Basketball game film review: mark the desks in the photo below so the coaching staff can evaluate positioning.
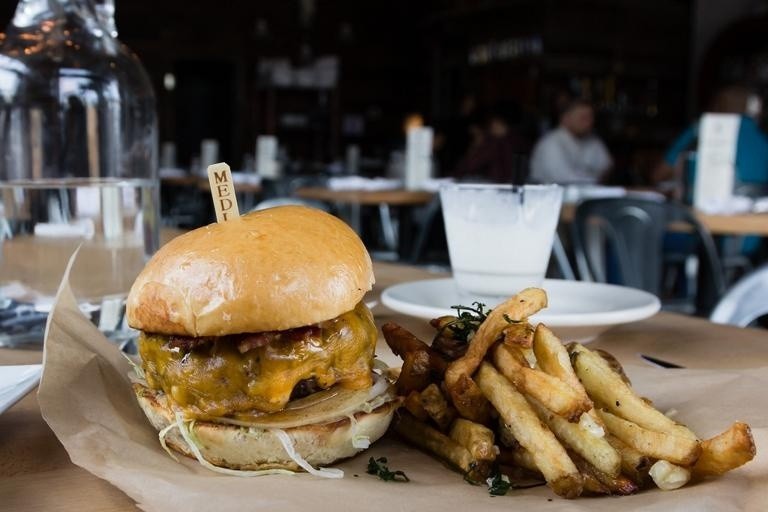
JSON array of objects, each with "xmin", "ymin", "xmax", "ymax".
[{"xmin": 0, "ymin": 172, "xmax": 768, "ymax": 512}]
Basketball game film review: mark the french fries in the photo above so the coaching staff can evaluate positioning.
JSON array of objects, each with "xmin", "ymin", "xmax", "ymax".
[{"xmin": 381, "ymin": 285, "xmax": 757, "ymax": 499}]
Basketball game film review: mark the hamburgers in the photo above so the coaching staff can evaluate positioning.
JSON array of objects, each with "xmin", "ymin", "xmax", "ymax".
[{"xmin": 126, "ymin": 161, "xmax": 394, "ymax": 471}]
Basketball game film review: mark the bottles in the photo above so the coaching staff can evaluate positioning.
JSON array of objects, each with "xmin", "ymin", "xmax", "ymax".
[{"xmin": 0, "ymin": 0, "xmax": 165, "ymax": 347}]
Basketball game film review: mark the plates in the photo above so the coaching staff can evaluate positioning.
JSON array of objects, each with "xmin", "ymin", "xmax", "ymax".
[{"xmin": 380, "ymin": 275, "xmax": 664, "ymax": 348}]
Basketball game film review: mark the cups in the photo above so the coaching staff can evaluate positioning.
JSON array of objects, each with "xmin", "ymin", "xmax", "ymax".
[{"xmin": 440, "ymin": 181, "xmax": 564, "ymax": 299}]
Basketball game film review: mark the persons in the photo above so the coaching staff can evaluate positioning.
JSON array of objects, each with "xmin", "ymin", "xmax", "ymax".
[
  {"xmin": 527, "ymin": 98, "xmax": 616, "ymax": 280},
  {"xmin": 654, "ymin": 87, "xmax": 768, "ymax": 320}
]
